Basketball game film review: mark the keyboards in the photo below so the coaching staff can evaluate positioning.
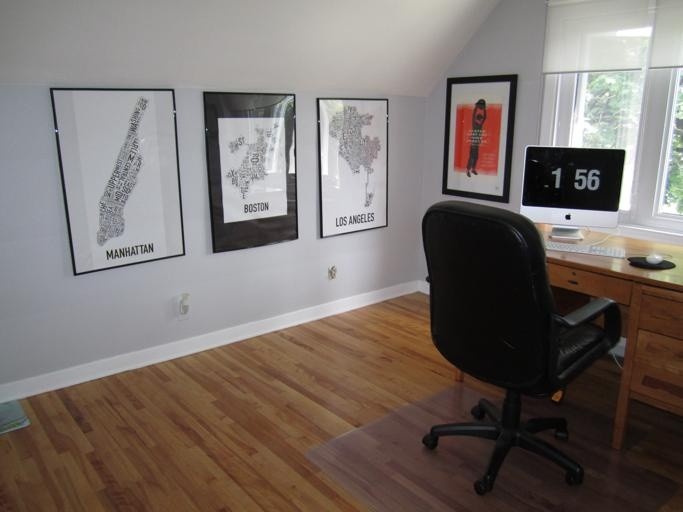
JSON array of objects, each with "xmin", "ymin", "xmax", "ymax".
[{"xmin": 542, "ymin": 240, "xmax": 626, "ymax": 260}]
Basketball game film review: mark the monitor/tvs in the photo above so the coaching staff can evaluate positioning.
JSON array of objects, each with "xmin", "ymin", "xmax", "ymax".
[{"xmin": 522, "ymin": 142, "xmax": 626, "ymax": 241}]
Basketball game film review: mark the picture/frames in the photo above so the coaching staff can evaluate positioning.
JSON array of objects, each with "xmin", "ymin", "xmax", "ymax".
[
  {"xmin": 317, "ymin": 98, "xmax": 389, "ymax": 239},
  {"xmin": 203, "ymin": 91, "xmax": 299, "ymax": 253},
  {"xmin": 49, "ymin": 87, "xmax": 186, "ymax": 275},
  {"xmin": 441, "ymin": 72, "xmax": 518, "ymax": 203}
]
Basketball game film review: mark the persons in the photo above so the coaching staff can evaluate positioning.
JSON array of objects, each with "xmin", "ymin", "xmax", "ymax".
[{"xmin": 466, "ymin": 99, "xmax": 487, "ymax": 177}]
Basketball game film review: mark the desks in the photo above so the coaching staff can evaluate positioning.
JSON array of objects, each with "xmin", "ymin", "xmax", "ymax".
[{"xmin": 530, "ymin": 221, "xmax": 683, "ymax": 452}]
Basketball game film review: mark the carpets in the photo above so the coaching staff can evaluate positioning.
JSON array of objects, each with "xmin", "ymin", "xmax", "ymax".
[{"xmin": 309, "ymin": 379, "xmax": 683, "ymax": 512}]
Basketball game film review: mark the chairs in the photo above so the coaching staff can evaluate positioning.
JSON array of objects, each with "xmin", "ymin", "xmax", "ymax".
[{"xmin": 423, "ymin": 200, "xmax": 622, "ymax": 496}]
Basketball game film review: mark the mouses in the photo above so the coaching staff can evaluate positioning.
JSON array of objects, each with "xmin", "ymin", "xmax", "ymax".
[{"xmin": 647, "ymin": 253, "xmax": 663, "ymax": 265}]
